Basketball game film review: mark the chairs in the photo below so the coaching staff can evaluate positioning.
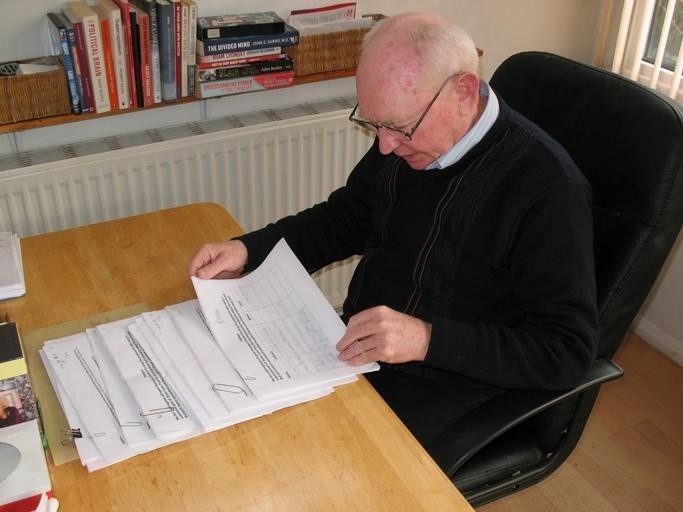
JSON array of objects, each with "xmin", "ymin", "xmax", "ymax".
[{"xmin": 308, "ymin": 52, "xmax": 683, "ymax": 508}]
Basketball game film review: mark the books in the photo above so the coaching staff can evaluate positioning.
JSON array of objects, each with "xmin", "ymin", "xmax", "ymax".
[
  {"xmin": 0, "ymin": 319, "xmax": 61, "ymax": 512},
  {"xmin": 46, "ymin": 2, "xmax": 301, "ymax": 117}
]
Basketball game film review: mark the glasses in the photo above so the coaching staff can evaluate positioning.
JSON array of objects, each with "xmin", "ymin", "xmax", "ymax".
[{"xmin": 348, "ymin": 70, "xmax": 464, "ymax": 146}]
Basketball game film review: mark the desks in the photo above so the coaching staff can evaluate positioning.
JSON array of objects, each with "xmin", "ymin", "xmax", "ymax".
[{"xmin": 0, "ymin": 204, "xmax": 477, "ymax": 512}]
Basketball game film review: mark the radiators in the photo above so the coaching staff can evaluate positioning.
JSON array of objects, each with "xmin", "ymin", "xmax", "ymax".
[{"xmin": 0, "ymin": 107, "xmax": 377, "ymax": 318}]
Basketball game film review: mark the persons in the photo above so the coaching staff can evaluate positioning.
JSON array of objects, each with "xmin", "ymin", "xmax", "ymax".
[{"xmin": 186, "ymin": 11, "xmax": 603, "ymax": 459}]
